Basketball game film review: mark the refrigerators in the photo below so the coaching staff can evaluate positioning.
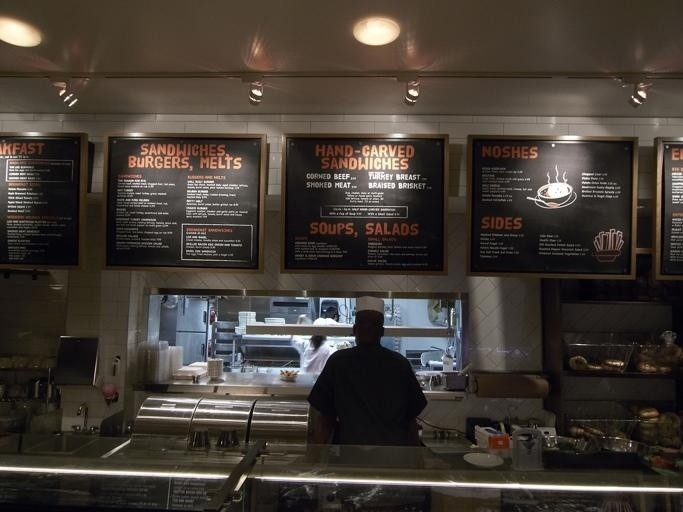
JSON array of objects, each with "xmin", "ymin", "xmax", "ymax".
[{"xmin": 174, "ymin": 299, "xmax": 212, "ymax": 365}]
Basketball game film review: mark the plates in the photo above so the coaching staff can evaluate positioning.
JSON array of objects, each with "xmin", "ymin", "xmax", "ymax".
[
  {"xmin": 208, "ymin": 359, "xmax": 224, "ymax": 380},
  {"xmin": 534, "ymin": 191, "xmax": 578, "ymax": 210},
  {"xmin": 463, "ymin": 452, "xmax": 504, "ymax": 469},
  {"xmin": 235, "ymin": 311, "xmax": 285, "ymax": 334}
]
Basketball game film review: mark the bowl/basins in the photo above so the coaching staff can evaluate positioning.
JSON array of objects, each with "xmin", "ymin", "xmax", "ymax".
[
  {"xmin": 537, "ymin": 183, "xmax": 573, "ymax": 205},
  {"xmin": 567, "ymin": 342, "xmax": 677, "ymax": 373},
  {"xmin": 280, "ymin": 372, "xmax": 297, "ymax": 381}
]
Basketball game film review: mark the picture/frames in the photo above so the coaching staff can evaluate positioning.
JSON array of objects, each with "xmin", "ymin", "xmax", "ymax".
[
  {"xmin": 466, "ymin": 133, "xmax": 639, "ymax": 278},
  {"xmin": 280, "ymin": 133, "xmax": 450, "ymax": 277},
  {"xmin": 650, "ymin": 137, "xmax": 683, "ymax": 281},
  {"xmin": 102, "ymin": 133, "xmax": 267, "ymax": 271},
  {"xmin": 0, "ymin": 131, "xmax": 91, "ymax": 270}
]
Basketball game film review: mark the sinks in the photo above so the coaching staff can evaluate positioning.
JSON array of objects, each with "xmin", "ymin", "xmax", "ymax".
[{"xmin": 22, "ymin": 433, "xmax": 97, "ymax": 457}]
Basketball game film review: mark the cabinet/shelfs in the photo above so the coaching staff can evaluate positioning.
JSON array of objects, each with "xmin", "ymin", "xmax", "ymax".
[
  {"xmin": 0, "ymin": 367, "xmax": 60, "ymax": 436},
  {"xmin": 539, "ymin": 277, "xmax": 683, "ymax": 434}
]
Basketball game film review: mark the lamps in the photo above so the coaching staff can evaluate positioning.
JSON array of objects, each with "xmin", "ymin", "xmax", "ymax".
[{"xmin": 0, "ymin": 69, "xmax": 683, "ymax": 108}]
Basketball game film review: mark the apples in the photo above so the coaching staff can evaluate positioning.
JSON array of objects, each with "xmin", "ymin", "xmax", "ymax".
[{"xmin": 101, "ymin": 383, "xmax": 115, "ymax": 397}]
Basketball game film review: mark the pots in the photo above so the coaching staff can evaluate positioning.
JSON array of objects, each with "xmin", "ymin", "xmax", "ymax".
[{"xmin": 541, "ymin": 426, "xmax": 647, "ymax": 463}]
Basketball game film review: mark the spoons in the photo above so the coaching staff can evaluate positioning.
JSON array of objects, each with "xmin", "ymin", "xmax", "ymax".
[{"xmin": 526, "ymin": 197, "xmax": 562, "ymax": 209}]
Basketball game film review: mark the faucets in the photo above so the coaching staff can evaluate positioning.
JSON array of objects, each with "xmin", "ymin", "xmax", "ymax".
[{"xmin": 77, "ymin": 403, "xmax": 89, "ymax": 433}]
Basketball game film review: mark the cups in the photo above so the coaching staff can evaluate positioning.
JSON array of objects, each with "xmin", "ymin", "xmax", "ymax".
[
  {"xmin": 216, "ymin": 426, "xmax": 240, "ymax": 449},
  {"xmin": 447, "ymin": 307, "xmax": 456, "ymax": 328},
  {"xmin": 188, "ymin": 426, "xmax": 211, "ymax": 450}
]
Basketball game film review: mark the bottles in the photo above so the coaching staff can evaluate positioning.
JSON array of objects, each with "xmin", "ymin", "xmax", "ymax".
[{"xmin": 442, "ymin": 342, "xmax": 456, "ymax": 372}]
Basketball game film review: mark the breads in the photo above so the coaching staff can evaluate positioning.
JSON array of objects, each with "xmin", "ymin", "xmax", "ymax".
[
  {"xmin": 569, "ymin": 344, "xmax": 682, "ymax": 374},
  {"xmin": 637, "ymin": 408, "xmax": 681, "ymax": 448}
]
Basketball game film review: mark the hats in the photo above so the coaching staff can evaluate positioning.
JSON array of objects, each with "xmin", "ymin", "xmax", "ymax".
[{"xmin": 356, "ymin": 295, "xmax": 384, "ymax": 315}]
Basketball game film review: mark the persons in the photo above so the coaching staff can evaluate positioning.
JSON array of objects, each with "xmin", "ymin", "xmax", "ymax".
[
  {"xmin": 290, "ymin": 301, "xmax": 338, "ymax": 371},
  {"xmin": 307, "ymin": 296, "xmax": 429, "ymax": 446}
]
